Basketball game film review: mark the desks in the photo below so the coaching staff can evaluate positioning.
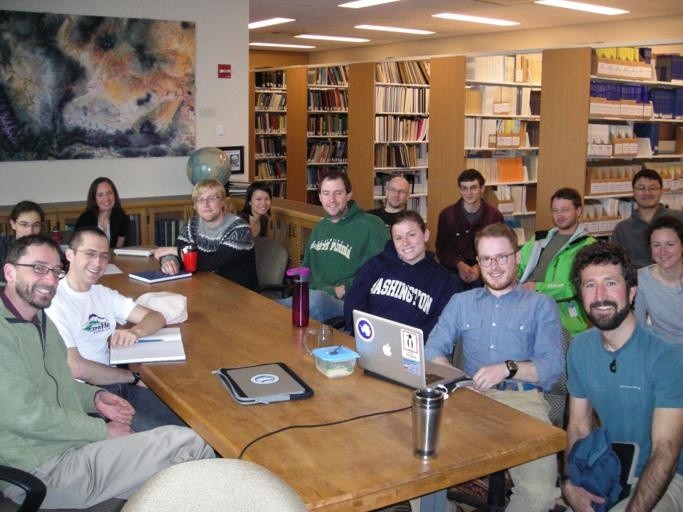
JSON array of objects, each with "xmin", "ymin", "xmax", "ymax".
[{"xmin": 59, "ymin": 245, "xmax": 569, "ymax": 511}]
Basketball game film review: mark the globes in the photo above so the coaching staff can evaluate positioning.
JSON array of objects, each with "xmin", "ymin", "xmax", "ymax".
[{"xmin": 186, "ymin": 146, "xmax": 231, "ymax": 186}]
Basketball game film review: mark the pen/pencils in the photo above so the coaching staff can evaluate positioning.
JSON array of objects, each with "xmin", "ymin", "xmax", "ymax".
[{"xmin": 136, "ymin": 339, "xmax": 163, "ymax": 342}]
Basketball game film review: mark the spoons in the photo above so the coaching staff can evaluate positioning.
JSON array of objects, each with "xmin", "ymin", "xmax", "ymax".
[{"xmin": 329, "ymin": 344, "xmax": 343, "ymax": 354}]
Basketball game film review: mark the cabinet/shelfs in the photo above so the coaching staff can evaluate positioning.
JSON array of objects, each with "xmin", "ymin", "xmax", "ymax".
[
  {"xmin": 427, "ymin": 49, "xmax": 542, "ymax": 255},
  {"xmin": 286, "ymin": 64, "xmax": 348, "ymax": 204},
  {"xmin": 535, "ymin": 46, "xmax": 682, "ymax": 248},
  {"xmin": 348, "ymin": 57, "xmax": 431, "ymax": 230},
  {"xmin": 250, "ymin": 69, "xmax": 286, "ymax": 200}
]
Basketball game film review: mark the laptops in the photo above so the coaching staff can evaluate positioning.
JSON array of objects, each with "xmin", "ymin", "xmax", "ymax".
[{"xmin": 351, "ymin": 308, "xmax": 466, "ymax": 391}]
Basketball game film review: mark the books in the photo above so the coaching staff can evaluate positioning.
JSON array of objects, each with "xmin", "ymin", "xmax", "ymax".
[
  {"xmin": 109, "ymin": 326, "xmax": 187, "ymax": 364},
  {"xmin": 155, "ymin": 215, "xmax": 181, "ymax": 248},
  {"xmin": 127, "ymin": 214, "xmax": 141, "ymax": 247},
  {"xmin": 129, "ymin": 270, "xmax": 192, "ymax": 284},
  {"xmin": 254, "ymin": 67, "xmax": 287, "ymax": 198},
  {"xmin": 226, "ymin": 181, "xmax": 251, "ymax": 196},
  {"xmin": 374, "ymin": 60, "xmax": 430, "ymax": 220},
  {"xmin": 464, "ymin": 53, "xmax": 540, "ymax": 243},
  {"xmin": 306, "ymin": 65, "xmax": 348, "ymax": 188}
]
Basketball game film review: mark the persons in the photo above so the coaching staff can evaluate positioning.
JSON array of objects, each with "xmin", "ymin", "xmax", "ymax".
[
  {"xmin": 367, "ymin": 176, "xmax": 426, "ymax": 235},
  {"xmin": 278, "ymin": 171, "xmax": 391, "ymax": 323},
  {"xmin": 0, "ymin": 233, "xmax": 216, "ymax": 510},
  {"xmin": 556, "ymin": 242, "xmax": 682, "ymax": 511},
  {"xmin": 155, "ymin": 178, "xmax": 260, "ymax": 292},
  {"xmin": 518, "ymin": 186, "xmax": 601, "ymax": 331},
  {"xmin": 43, "ymin": 227, "xmax": 190, "ymax": 431},
  {"xmin": 336, "ymin": 210, "xmax": 461, "ymax": 342},
  {"xmin": 432, "ymin": 170, "xmax": 509, "ymax": 288},
  {"xmin": 633, "ymin": 218, "xmax": 682, "ymax": 343},
  {"xmin": 422, "ymin": 222, "xmax": 567, "ymax": 511},
  {"xmin": 237, "ymin": 183, "xmax": 273, "ymax": 239},
  {"xmin": 0, "ymin": 200, "xmax": 45, "ymax": 282},
  {"xmin": 607, "ymin": 169, "xmax": 683, "ymax": 286},
  {"xmin": 75, "ymin": 177, "xmax": 129, "ymax": 248}
]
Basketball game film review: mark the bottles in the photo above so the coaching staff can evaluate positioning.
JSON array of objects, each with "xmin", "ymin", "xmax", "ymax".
[
  {"xmin": 286, "ymin": 267, "xmax": 311, "ymax": 327},
  {"xmin": 52, "ymin": 225, "xmax": 61, "ymax": 244}
]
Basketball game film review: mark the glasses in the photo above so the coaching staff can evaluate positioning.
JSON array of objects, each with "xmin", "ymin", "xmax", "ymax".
[
  {"xmin": 197, "ymin": 196, "xmax": 220, "ymax": 204},
  {"xmin": 478, "ymin": 252, "xmax": 515, "ymax": 267},
  {"xmin": 13, "ymin": 263, "xmax": 66, "ymax": 279},
  {"xmin": 15, "ymin": 221, "xmax": 42, "ymax": 227},
  {"xmin": 71, "ymin": 248, "xmax": 112, "ymax": 261}
]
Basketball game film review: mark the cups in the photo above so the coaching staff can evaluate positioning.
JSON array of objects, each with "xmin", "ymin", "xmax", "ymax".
[
  {"xmin": 181, "ymin": 244, "xmax": 198, "ymax": 273},
  {"xmin": 300, "ymin": 324, "xmax": 334, "ymax": 356},
  {"xmin": 411, "ymin": 389, "xmax": 445, "ymax": 462}
]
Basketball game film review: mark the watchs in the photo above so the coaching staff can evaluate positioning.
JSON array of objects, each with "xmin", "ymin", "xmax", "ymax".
[
  {"xmin": 130, "ymin": 372, "xmax": 141, "ymax": 384},
  {"xmin": 502, "ymin": 359, "xmax": 518, "ymax": 381}
]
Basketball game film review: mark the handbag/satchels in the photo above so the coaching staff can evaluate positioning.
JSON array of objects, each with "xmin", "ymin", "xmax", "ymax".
[{"xmin": 567, "ymin": 426, "xmax": 623, "ymax": 512}]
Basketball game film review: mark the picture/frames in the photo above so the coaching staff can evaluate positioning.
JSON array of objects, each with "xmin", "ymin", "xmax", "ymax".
[{"xmin": 214, "ymin": 147, "xmax": 244, "ymax": 175}]
